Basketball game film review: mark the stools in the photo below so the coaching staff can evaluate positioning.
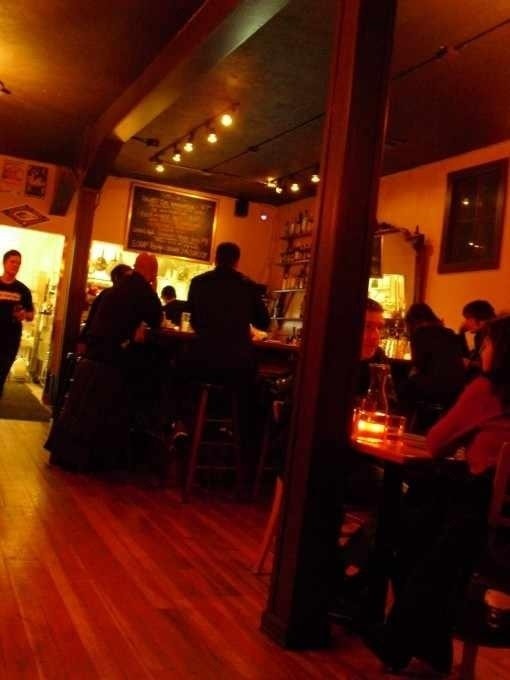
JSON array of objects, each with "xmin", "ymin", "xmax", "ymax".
[{"xmin": 47, "ymin": 351, "xmax": 288, "ymax": 500}]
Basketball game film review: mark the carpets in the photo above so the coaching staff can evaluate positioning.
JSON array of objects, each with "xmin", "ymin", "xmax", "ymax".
[{"xmin": 0, "ymin": 381, "xmax": 51, "ymax": 421}]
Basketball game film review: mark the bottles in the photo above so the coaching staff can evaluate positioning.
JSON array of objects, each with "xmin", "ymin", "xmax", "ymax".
[{"xmin": 355, "ymin": 363, "xmax": 390, "ymax": 445}]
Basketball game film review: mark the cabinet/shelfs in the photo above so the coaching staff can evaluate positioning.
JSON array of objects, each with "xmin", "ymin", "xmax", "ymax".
[
  {"xmin": 369, "ymin": 232, "xmax": 416, "ymax": 306},
  {"xmin": 269, "ymin": 232, "xmax": 312, "ymax": 320}
]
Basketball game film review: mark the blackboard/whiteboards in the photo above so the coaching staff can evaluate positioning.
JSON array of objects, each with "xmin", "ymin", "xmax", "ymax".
[{"xmin": 124, "ymin": 181, "xmax": 217, "ymax": 265}]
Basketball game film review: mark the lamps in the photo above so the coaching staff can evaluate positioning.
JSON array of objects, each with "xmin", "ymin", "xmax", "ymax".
[
  {"xmin": 148, "ymin": 103, "xmax": 240, "ymax": 173},
  {"xmin": 133, "ymin": 136, "xmax": 160, "ymax": 147},
  {"xmin": 266, "ymin": 160, "xmax": 320, "ymax": 193}
]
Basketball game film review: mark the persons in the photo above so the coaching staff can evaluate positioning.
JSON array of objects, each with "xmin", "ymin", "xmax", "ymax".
[{"xmin": 0, "ymin": 250, "xmax": 34, "ymax": 394}]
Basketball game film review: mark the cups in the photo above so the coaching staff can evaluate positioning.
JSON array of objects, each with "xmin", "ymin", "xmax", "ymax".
[
  {"xmin": 387, "ymin": 415, "xmax": 407, "ymax": 442},
  {"xmin": 180, "ymin": 312, "xmax": 191, "ymax": 332}
]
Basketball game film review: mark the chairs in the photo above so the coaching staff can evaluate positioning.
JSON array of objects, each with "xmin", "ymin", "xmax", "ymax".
[{"xmin": 340, "ymin": 395, "xmax": 510, "ymax": 680}]
